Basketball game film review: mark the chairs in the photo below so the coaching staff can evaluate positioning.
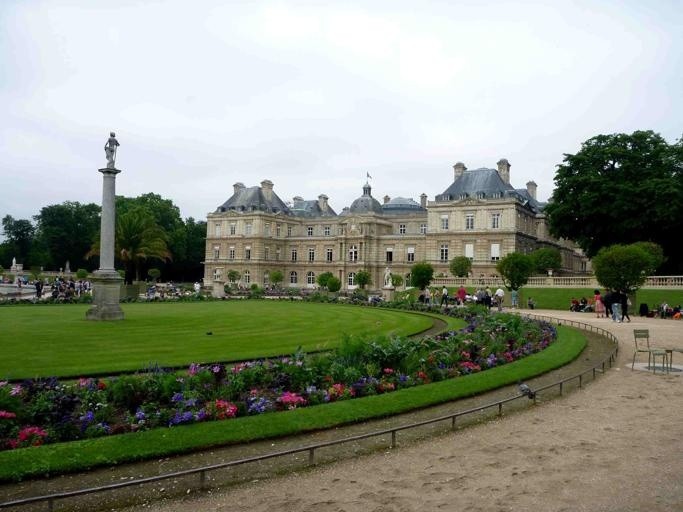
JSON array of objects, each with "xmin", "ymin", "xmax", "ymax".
[{"xmin": 631, "ymin": 328, "xmax": 669, "ymax": 375}]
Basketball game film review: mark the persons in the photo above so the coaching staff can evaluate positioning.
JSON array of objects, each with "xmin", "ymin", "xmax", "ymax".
[
  {"xmin": 145, "ymin": 278, "xmax": 192, "ymax": 302},
  {"xmin": 313, "ymin": 285, "xmax": 327, "ymax": 291},
  {"xmin": 400, "ymin": 292, "xmax": 411, "ymax": 305},
  {"xmin": 511, "ymin": 287, "xmax": 524, "ymax": 310},
  {"xmin": 336, "ymin": 291, "xmax": 349, "ymax": 297},
  {"xmin": 238, "ymin": 282, "xmax": 248, "ymax": 291},
  {"xmin": 265, "ymin": 283, "xmax": 294, "ymax": 298},
  {"xmin": 368, "ymin": 290, "xmax": 387, "ymax": 306},
  {"xmin": 193, "ymin": 281, "xmax": 201, "ymax": 293},
  {"xmin": 300, "ymin": 286, "xmax": 305, "ymax": 298},
  {"xmin": 32, "ymin": 270, "xmax": 90, "ymax": 304},
  {"xmin": 416, "ymin": 282, "xmax": 505, "ymax": 311},
  {"xmin": 569, "ymin": 287, "xmax": 632, "ymax": 323},
  {"xmin": 0, "ymin": 274, "xmax": 29, "ymax": 291},
  {"xmin": 104, "ymin": 132, "xmax": 120, "ymax": 169},
  {"xmin": 527, "ymin": 297, "xmax": 535, "ymax": 310},
  {"xmin": 652, "ymin": 299, "xmax": 682, "ymax": 320},
  {"xmin": 351, "ymin": 291, "xmax": 359, "ymax": 305}
]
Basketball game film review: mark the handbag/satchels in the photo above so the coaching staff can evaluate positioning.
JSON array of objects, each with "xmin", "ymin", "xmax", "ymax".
[
  {"xmin": 600, "ymin": 295, "xmax": 605, "ymax": 304},
  {"xmin": 626, "ymin": 296, "xmax": 632, "ymax": 306}
]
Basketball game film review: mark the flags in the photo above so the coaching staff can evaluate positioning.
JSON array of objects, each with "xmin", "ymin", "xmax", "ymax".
[{"xmin": 367, "ymin": 172, "xmax": 372, "ymax": 180}]
